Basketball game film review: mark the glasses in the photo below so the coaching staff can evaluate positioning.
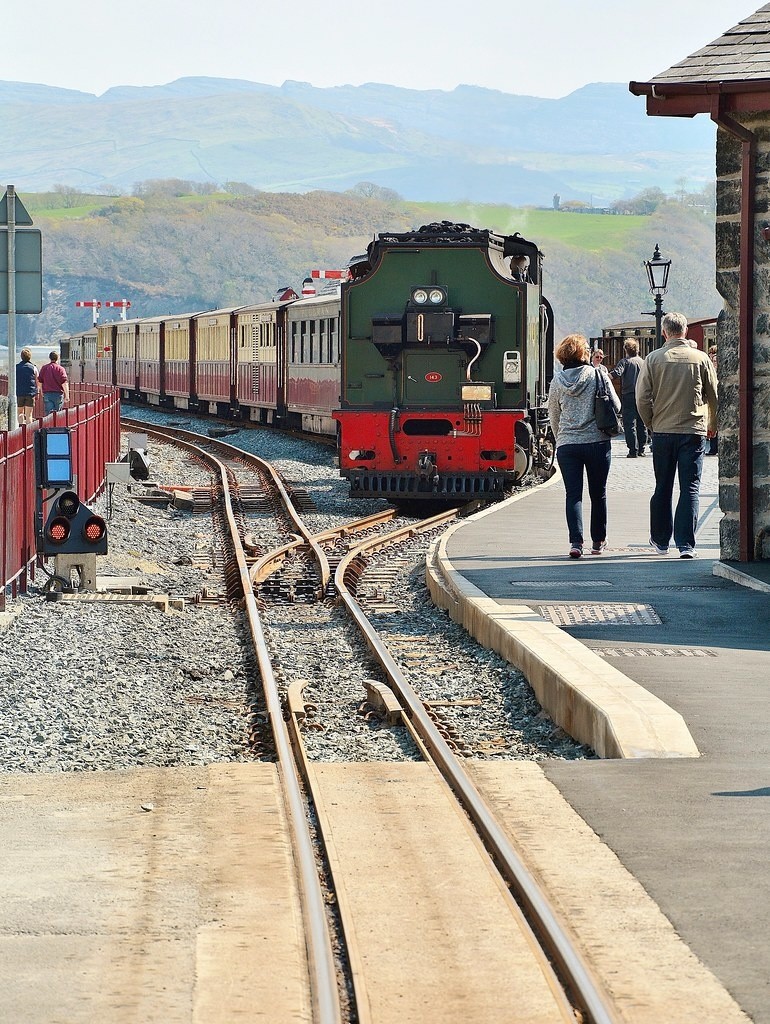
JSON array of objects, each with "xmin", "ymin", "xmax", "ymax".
[{"xmin": 594, "ymin": 355, "xmax": 604, "ymax": 359}]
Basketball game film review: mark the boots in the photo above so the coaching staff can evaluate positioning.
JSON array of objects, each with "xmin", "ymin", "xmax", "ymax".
[{"xmin": 705, "ymin": 437, "xmax": 718, "ymax": 455}]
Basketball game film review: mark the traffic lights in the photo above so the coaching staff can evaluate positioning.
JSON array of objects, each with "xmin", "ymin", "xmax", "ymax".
[{"xmin": 43, "ymin": 490, "xmax": 108, "ymax": 555}]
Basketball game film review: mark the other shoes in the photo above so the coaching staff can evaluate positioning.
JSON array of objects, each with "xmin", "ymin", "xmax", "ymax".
[
  {"xmin": 626, "ymin": 452, "xmax": 638, "ymax": 458},
  {"xmin": 638, "ymin": 452, "xmax": 646, "ymax": 457}
]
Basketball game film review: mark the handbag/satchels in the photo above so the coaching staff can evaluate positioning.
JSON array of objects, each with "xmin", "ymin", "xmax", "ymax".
[{"xmin": 593, "ymin": 367, "xmax": 624, "ymax": 437}]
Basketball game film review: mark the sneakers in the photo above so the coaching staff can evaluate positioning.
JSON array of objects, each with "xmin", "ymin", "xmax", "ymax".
[
  {"xmin": 649, "ymin": 537, "xmax": 669, "ymax": 554},
  {"xmin": 591, "ymin": 539, "xmax": 607, "ymax": 554},
  {"xmin": 569, "ymin": 541, "xmax": 583, "ymax": 559},
  {"xmin": 678, "ymin": 545, "xmax": 698, "ymax": 558}
]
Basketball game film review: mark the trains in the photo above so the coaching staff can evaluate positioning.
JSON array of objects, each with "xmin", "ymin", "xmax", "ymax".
[
  {"xmin": 589, "ymin": 315, "xmax": 717, "ymax": 417},
  {"xmin": 67, "ymin": 221, "xmax": 559, "ymax": 506}
]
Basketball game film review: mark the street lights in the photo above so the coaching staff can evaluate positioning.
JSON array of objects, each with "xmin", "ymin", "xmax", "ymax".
[{"xmin": 642, "ymin": 243, "xmax": 672, "ymax": 350}]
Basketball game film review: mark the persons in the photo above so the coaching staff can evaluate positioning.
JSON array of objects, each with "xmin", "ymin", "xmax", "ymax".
[
  {"xmin": 16, "ymin": 349, "xmax": 39, "ymax": 423},
  {"xmin": 608, "ymin": 338, "xmax": 649, "ymax": 458},
  {"xmin": 548, "ymin": 335, "xmax": 621, "ymax": 558},
  {"xmin": 635, "ymin": 312, "xmax": 718, "ymax": 558},
  {"xmin": 37, "ymin": 351, "xmax": 70, "ymax": 416}
]
